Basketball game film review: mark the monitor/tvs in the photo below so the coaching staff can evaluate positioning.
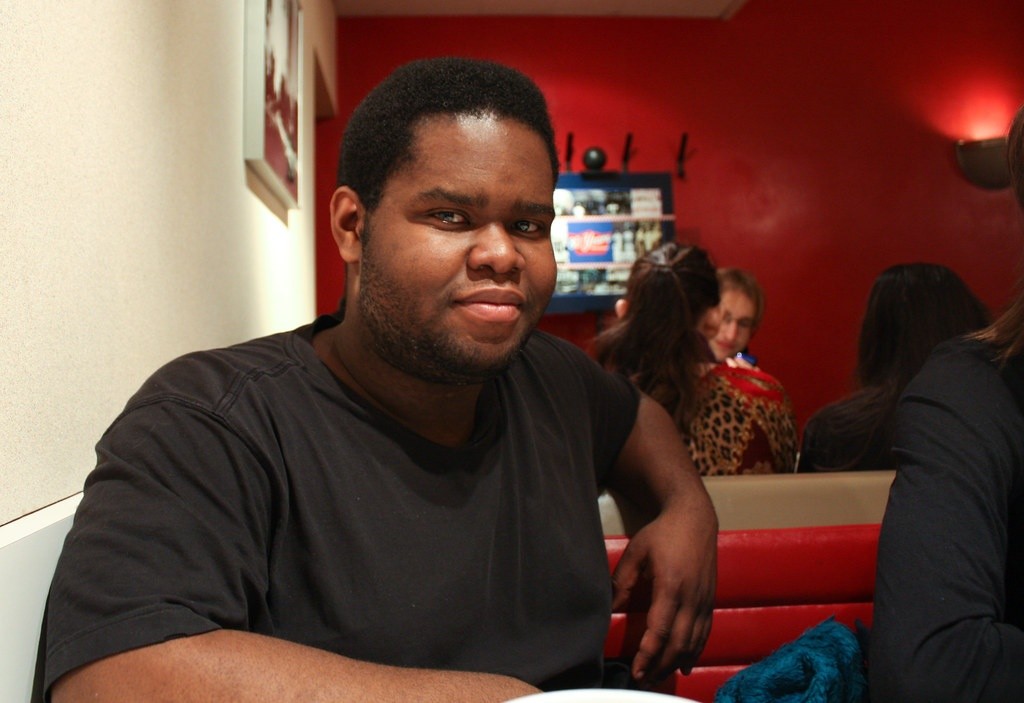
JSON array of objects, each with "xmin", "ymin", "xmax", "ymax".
[{"xmin": 541, "ymin": 171, "xmax": 675, "ymax": 313}]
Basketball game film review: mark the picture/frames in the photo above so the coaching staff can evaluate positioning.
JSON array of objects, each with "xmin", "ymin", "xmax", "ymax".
[{"xmin": 242, "ymin": 0, "xmax": 304, "ymax": 210}]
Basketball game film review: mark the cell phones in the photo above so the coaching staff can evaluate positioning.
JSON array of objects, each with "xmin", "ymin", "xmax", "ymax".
[{"xmin": 734, "ymin": 350, "xmax": 758, "ymax": 367}]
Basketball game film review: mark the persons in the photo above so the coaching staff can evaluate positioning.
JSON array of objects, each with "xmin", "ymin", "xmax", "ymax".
[
  {"xmin": 588, "ymin": 243, "xmax": 797, "ymax": 476},
  {"xmin": 29, "ymin": 59, "xmax": 720, "ymax": 703},
  {"xmin": 801, "ymin": 263, "xmax": 989, "ymax": 475},
  {"xmin": 709, "ymin": 267, "xmax": 762, "ymax": 369},
  {"xmin": 864, "ymin": 108, "xmax": 1023, "ymax": 702}
]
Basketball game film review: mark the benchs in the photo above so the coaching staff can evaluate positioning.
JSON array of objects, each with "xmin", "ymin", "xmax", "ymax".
[{"xmin": 579, "ymin": 471, "xmax": 898, "ymax": 702}]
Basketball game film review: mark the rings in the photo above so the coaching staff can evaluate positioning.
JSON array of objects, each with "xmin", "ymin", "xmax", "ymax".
[{"xmin": 740, "ymin": 361, "xmax": 746, "ymax": 366}]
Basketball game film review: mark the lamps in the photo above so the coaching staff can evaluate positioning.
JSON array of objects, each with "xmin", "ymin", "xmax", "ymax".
[{"xmin": 955, "ymin": 136, "xmax": 1011, "ymax": 189}]
